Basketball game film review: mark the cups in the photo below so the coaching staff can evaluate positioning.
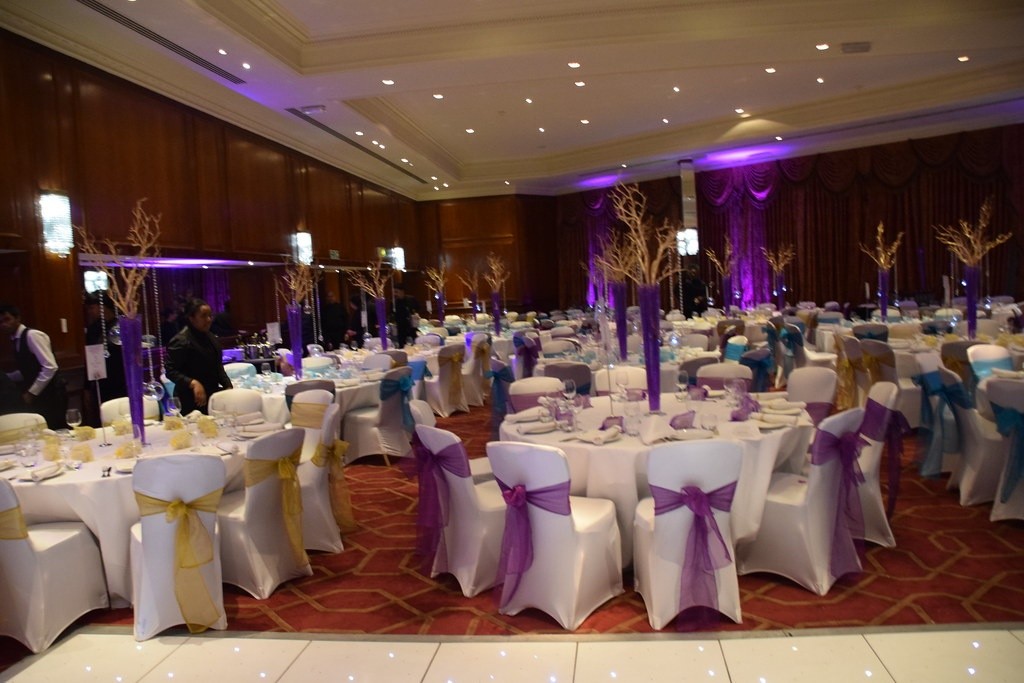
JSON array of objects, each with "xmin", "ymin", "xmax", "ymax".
[
  {"xmin": 687, "ymin": 391, "xmax": 702, "ymax": 413},
  {"xmin": 119, "ymin": 400, "xmax": 132, "ymax": 421},
  {"xmin": 723, "ymin": 371, "xmax": 739, "ymax": 394},
  {"xmin": 547, "ymin": 388, "xmax": 560, "ymax": 402},
  {"xmin": 562, "ymin": 379, "xmax": 576, "ymax": 400},
  {"xmin": 66, "ymin": 409, "xmax": 82, "ymax": 429},
  {"xmin": 167, "ymin": 397, "xmax": 182, "ymax": 416},
  {"xmin": 212, "ymin": 396, "xmax": 226, "ymax": 416}
]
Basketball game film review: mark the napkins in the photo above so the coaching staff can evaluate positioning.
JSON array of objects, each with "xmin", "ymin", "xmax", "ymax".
[
  {"xmin": 503, "ymin": 411, "xmax": 543, "ymax": 422},
  {"xmin": 30, "ymin": 459, "xmax": 64, "ymax": 482},
  {"xmin": 215, "ymin": 440, "xmax": 239, "ymax": 455},
  {"xmin": 237, "ymin": 423, "xmax": 285, "ymax": 434},
  {"xmin": 593, "ymin": 423, "xmax": 622, "ymax": 446},
  {"xmin": 766, "ymin": 399, "xmax": 807, "ymax": 410},
  {"xmin": 0, "ymin": 457, "xmax": 18, "ymax": 474},
  {"xmin": 237, "ymin": 410, "xmax": 262, "ymax": 424},
  {"xmin": 750, "ymin": 410, "xmax": 799, "ymax": 425}
]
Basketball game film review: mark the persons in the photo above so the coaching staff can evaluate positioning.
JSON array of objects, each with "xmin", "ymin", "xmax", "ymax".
[
  {"xmin": 297, "ymin": 292, "xmax": 379, "ymax": 357},
  {"xmin": 84, "ymin": 293, "xmax": 130, "ymax": 402},
  {"xmin": 0, "ymin": 301, "xmax": 69, "ymax": 429},
  {"xmin": 164, "ymin": 298, "xmax": 233, "ymax": 417},
  {"xmin": 211, "ymin": 299, "xmax": 237, "ymax": 337},
  {"xmin": 676, "ymin": 263, "xmax": 708, "ymax": 318},
  {"xmin": 390, "ymin": 283, "xmax": 422, "ymax": 349},
  {"xmin": 161, "ymin": 306, "xmax": 178, "ymax": 345}
]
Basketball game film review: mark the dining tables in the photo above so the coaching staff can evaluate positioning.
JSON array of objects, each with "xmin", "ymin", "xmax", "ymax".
[
  {"xmin": 237, "ymin": 364, "xmax": 383, "ymax": 423},
  {"xmin": 0, "ymin": 418, "xmax": 294, "ymax": 608},
  {"xmin": 500, "ymin": 387, "xmax": 814, "ymax": 568}
]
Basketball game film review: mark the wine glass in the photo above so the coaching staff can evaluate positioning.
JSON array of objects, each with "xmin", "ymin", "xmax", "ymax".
[
  {"xmin": 125, "ymin": 423, "xmax": 143, "ymax": 460},
  {"xmin": 225, "ymin": 412, "xmax": 236, "ymax": 437},
  {"xmin": 675, "ymin": 370, "xmax": 689, "ymax": 400},
  {"xmin": 623, "ymin": 399, "xmax": 640, "ymax": 435},
  {"xmin": 187, "ymin": 415, "xmax": 202, "ymax": 452},
  {"xmin": 55, "ymin": 429, "xmax": 72, "ymax": 468},
  {"xmin": 615, "ymin": 372, "xmax": 629, "ymax": 401},
  {"xmin": 24, "ymin": 418, "xmax": 41, "ymax": 448}
]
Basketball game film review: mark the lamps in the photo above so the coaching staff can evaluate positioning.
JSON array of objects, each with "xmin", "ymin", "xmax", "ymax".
[
  {"xmin": 296, "ymin": 231, "xmax": 406, "ymax": 271},
  {"xmin": 684, "ymin": 227, "xmax": 700, "ymax": 256},
  {"xmin": 37, "ymin": 188, "xmax": 75, "ymax": 259}
]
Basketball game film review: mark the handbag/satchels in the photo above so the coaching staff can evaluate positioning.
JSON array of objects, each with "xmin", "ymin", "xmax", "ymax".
[{"xmin": 410, "ymin": 312, "xmax": 421, "ymax": 328}]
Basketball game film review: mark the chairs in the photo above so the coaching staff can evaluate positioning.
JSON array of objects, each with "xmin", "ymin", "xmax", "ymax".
[
  {"xmin": 0, "ymin": 389, "xmax": 358, "ymax": 652},
  {"xmin": 0, "ymin": 297, "xmax": 1024, "ymax": 655}
]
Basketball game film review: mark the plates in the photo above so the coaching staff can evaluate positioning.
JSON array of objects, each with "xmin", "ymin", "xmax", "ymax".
[
  {"xmin": 238, "ymin": 430, "xmax": 275, "ymax": 438},
  {"xmin": 233, "ymin": 419, "xmax": 264, "ymax": 425},
  {"xmin": 675, "ymin": 429, "xmax": 714, "ymax": 440},
  {"xmin": 525, "ymin": 427, "xmax": 555, "ymax": 433},
  {"xmin": 743, "ymin": 419, "xmax": 784, "ymax": 429},
  {"xmin": 761, "ymin": 405, "xmax": 800, "ymax": 416},
  {"xmin": 116, "ymin": 457, "xmax": 136, "ymax": 472},
  {"xmin": 189, "ymin": 446, "xmax": 236, "ymax": 455},
  {"xmin": 200, "ymin": 445, "xmax": 231, "ymax": 455},
  {"xmin": 18, "ymin": 468, "xmax": 65, "ymax": 480},
  {"xmin": 578, "ymin": 430, "xmax": 623, "ymax": 442}
]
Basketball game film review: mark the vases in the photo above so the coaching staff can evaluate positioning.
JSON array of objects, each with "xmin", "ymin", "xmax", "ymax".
[
  {"xmin": 964, "ymin": 264, "xmax": 982, "ymax": 342},
  {"xmin": 119, "ymin": 316, "xmax": 150, "ymax": 446},
  {"xmin": 285, "ymin": 306, "xmax": 307, "ymax": 382},
  {"xmin": 378, "ymin": 299, "xmax": 388, "ymax": 353},
  {"xmin": 611, "ymin": 283, "xmax": 631, "ymax": 358},
  {"xmin": 489, "ymin": 291, "xmax": 504, "ymax": 335},
  {"xmin": 775, "ymin": 276, "xmax": 786, "ymax": 312},
  {"xmin": 637, "ymin": 285, "xmax": 663, "ymax": 412},
  {"xmin": 881, "ymin": 267, "xmax": 890, "ymax": 322}
]
